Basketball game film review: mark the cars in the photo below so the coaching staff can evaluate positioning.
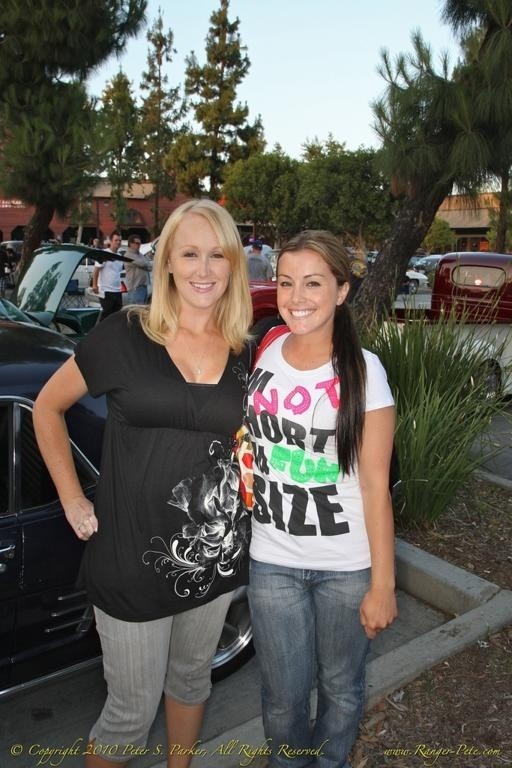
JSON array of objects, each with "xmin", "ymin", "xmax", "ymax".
[
  {"xmin": 0, "ymin": 235, "xmax": 510, "ymax": 414},
  {"xmin": 1, "ymin": 322, "xmax": 403, "ymax": 707}
]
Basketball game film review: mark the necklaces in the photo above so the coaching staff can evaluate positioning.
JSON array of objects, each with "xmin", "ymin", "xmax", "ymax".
[{"xmin": 177, "ymin": 321, "xmax": 218, "ymax": 375}]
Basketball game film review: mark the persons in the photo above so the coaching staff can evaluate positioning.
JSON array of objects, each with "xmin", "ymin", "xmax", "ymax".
[
  {"xmin": 0, "ymin": 230, "xmax": 18, "ymax": 297},
  {"xmin": 32, "ymin": 200, "xmax": 258, "ymax": 768},
  {"xmin": 92, "ymin": 229, "xmax": 122, "ymax": 327},
  {"xmin": 247, "ymin": 240, "xmax": 273, "ymax": 282},
  {"xmin": 92, "ymin": 238, "xmax": 100, "ymax": 248},
  {"xmin": 247, "ymin": 230, "xmax": 399, "ymax": 768},
  {"xmin": 123, "ymin": 234, "xmax": 153, "ymax": 305}
]
